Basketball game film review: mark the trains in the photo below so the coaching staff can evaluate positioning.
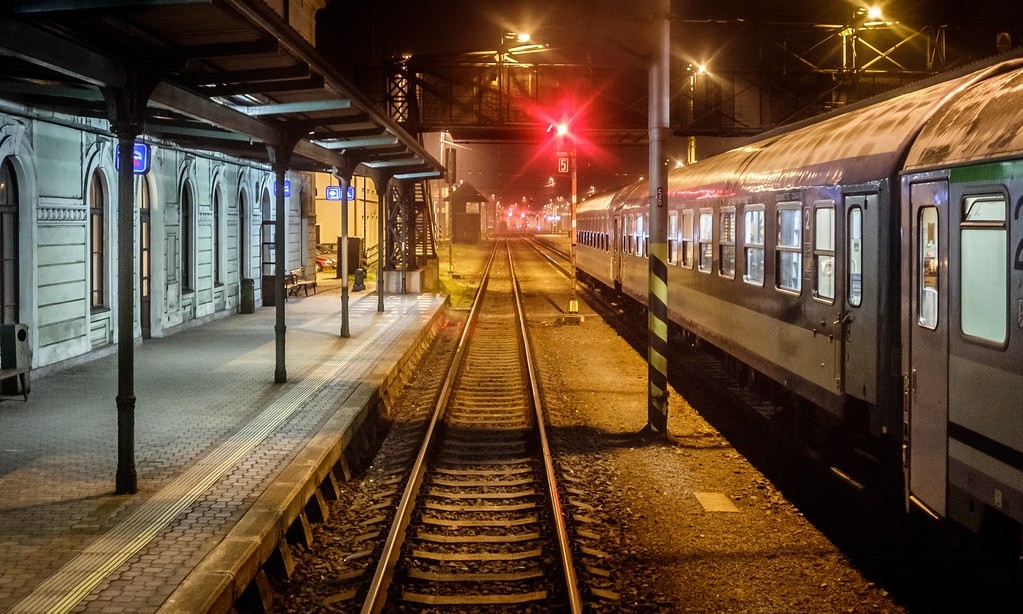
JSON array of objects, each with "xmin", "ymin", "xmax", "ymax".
[{"xmin": 576, "ymin": 47, "xmax": 1022, "ymax": 559}]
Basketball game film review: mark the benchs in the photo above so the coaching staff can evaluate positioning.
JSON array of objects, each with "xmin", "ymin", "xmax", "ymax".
[
  {"xmin": 290, "ymin": 266, "xmax": 318, "ymax": 297},
  {"xmin": 285, "ymin": 274, "xmax": 299, "ymax": 302}
]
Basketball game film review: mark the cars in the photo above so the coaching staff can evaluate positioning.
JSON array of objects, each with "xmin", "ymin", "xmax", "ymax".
[{"xmin": 315, "ymin": 244, "xmax": 339, "ymax": 271}]
